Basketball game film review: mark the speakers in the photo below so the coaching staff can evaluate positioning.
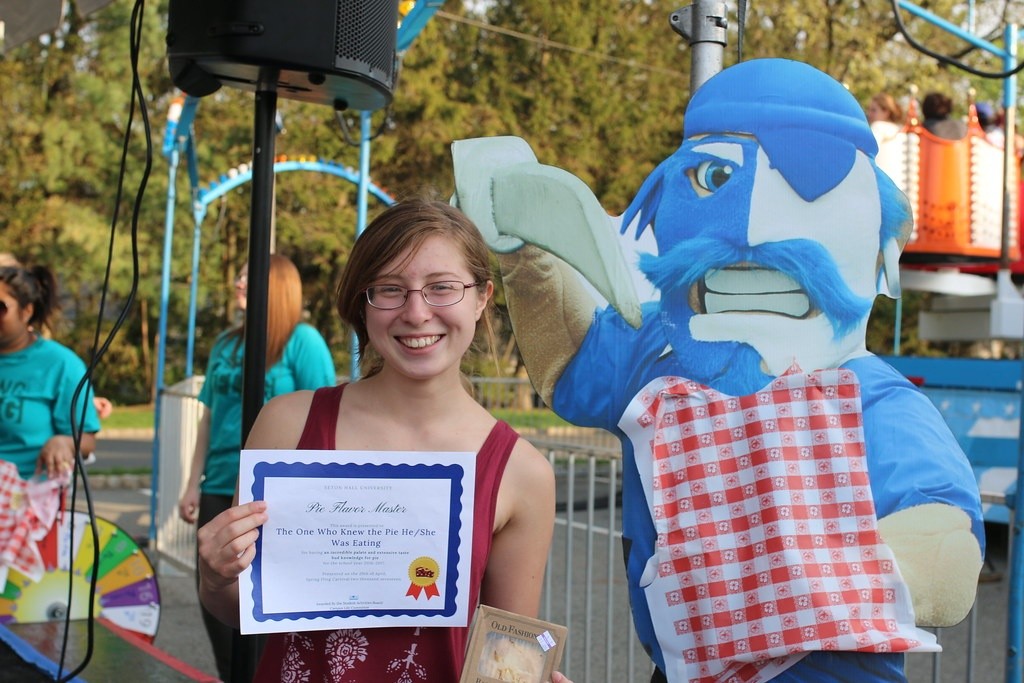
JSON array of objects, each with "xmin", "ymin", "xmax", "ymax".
[{"xmin": 166, "ymin": 0, "xmax": 399, "ymax": 111}]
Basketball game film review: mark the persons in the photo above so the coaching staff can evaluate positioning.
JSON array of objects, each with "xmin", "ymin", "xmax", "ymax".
[
  {"xmin": 0, "ymin": 252, "xmax": 112, "ymax": 584},
  {"xmin": 178, "ymin": 199, "xmax": 571, "ymax": 682},
  {"xmin": 450, "ymin": 57, "xmax": 984, "ymax": 683},
  {"xmin": 868, "ymin": 93, "xmax": 1024, "ymax": 163}
]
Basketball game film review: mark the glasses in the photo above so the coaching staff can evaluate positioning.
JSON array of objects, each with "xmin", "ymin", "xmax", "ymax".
[
  {"xmin": 0, "ymin": 300, "xmax": 9, "ymax": 315},
  {"xmin": 365, "ymin": 280, "xmax": 479, "ymax": 310}
]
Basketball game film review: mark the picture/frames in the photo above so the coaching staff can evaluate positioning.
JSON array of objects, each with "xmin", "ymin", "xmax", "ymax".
[{"xmin": 459, "ymin": 604, "xmax": 569, "ymax": 683}]
